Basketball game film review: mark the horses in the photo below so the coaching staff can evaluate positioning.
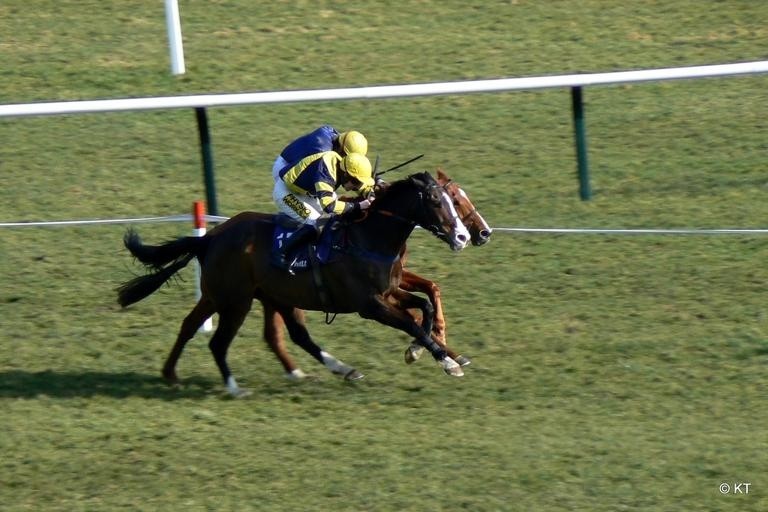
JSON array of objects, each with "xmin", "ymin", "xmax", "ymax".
[
  {"xmin": 123, "ymin": 170, "xmax": 472, "ymax": 399},
  {"xmin": 112, "ymin": 166, "xmax": 494, "ymax": 383}
]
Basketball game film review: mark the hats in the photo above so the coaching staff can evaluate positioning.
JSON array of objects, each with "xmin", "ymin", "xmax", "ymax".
[
  {"xmin": 337, "ymin": 131, "xmax": 368, "ymax": 154},
  {"xmin": 341, "ymin": 156, "xmax": 375, "ymax": 187}
]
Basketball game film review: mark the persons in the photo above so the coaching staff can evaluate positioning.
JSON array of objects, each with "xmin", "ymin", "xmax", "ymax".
[
  {"xmin": 273, "ymin": 151, "xmax": 375, "ymax": 270},
  {"xmin": 273, "ymin": 126, "xmax": 392, "ymax": 202}
]
